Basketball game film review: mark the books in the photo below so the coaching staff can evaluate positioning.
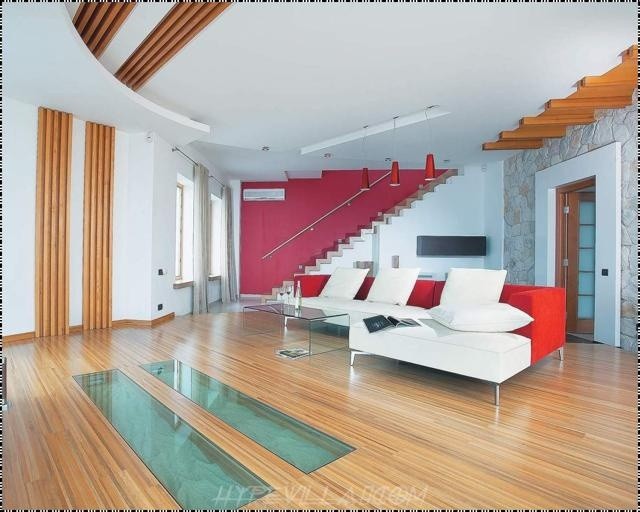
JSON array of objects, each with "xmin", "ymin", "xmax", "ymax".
[{"xmin": 363, "ymin": 314, "xmax": 421, "ymax": 334}]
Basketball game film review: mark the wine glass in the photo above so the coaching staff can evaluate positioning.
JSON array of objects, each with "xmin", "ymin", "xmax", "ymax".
[{"xmin": 279, "ymin": 286, "xmax": 292, "ymax": 306}]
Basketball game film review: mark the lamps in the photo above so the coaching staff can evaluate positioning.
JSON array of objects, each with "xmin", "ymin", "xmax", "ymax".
[
  {"xmin": 424, "ymin": 105, "xmax": 436, "ymax": 180},
  {"xmin": 360, "ymin": 126, "xmax": 370, "ymax": 191},
  {"xmin": 389, "ymin": 117, "xmax": 400, "ymax": 186}
]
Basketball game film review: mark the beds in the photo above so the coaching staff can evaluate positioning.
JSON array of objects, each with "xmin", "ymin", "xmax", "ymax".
[{"xmin": 243, "ymin": 304, "xmax": 350, "ymax": 356}]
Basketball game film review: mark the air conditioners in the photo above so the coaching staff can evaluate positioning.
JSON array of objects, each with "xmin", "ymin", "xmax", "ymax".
[{"xmin": 243, "ymin": 188, "xmax": 286, "ymax": 202}]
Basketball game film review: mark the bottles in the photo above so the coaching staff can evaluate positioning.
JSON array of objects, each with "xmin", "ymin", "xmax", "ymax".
[{"xmin": 295, "ymin": 281, "xmax": 301, "ymax": 310}]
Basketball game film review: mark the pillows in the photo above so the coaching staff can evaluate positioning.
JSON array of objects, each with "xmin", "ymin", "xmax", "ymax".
[
  {"xmin": 428, "ymin": 302, "xmax": 535, "ymax": 333},
  {"xmin": 364, "ymin": 267, "xmax": 419, "ymax": 306},
  {"xmin": 318, "ymin": 266, "xmax": 370, "ymax": 300},
  {"xmin": 439, "ymin": 267, "xmax": 508, "ymax": 304}
]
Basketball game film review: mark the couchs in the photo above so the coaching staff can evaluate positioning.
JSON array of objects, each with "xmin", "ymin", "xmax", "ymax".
[{"xmin": 294, "ymin": 275, "xmax": 567, "ymax": 406}]
